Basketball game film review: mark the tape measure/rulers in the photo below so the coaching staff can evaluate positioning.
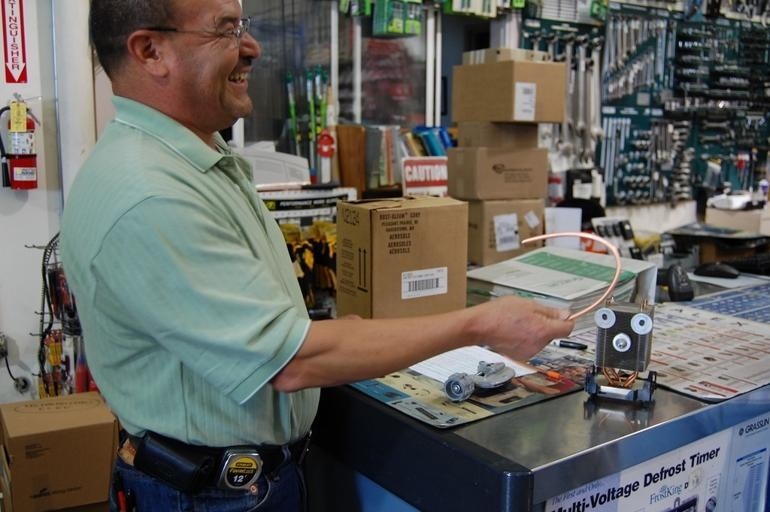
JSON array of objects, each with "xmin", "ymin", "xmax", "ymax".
[{"xmin": 214, "ymin": 447, "xmax": 266, "ymax": 495}]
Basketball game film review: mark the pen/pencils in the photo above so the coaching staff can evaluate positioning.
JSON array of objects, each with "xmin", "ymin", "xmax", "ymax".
[{"xmin": 549, "ymin": 339, "xmax": 588, "ymax": 350}]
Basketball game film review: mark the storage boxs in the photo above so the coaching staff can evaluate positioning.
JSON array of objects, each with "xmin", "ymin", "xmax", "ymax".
[
  {"xmin": 0, "ymin": 391, "xmax": 120, "ymax": 512},
  {"xmin": 447, "ymin": 147, "xmax": 548, "ymax": 200},
  {"xmin": 467, "ymin": 200, "xmax": 544, "ymax": 267},
  {"xmin": 450, "ymin": 60, "xmax": 567, "ymax": 124},
  {"xmin": 456, "ymin": 122, "xmax": 538, "ymax": 148},
  {"xmin": 334, "ymin": 196, "xmax": 468, "ymax": 319}
]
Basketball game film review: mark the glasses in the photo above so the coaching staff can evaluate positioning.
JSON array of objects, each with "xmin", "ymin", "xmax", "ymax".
[{"xmin": 142, "ymin": 17, "xmax": 252, "ymax": 47}]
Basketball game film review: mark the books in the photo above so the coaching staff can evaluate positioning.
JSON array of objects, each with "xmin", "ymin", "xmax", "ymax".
[{"xmin": 466, "ymin": 244, "xmax": 658, "ymax": 340}]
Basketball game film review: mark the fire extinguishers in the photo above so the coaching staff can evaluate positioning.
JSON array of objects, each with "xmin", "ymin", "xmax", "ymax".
[{"xmin": 0, "ymin": 92, "xmax": 41, "ymax": 190}]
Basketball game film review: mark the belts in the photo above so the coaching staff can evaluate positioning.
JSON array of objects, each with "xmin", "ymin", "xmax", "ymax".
[{"xmin": 118, "ymin": 428, "xmax": 312, "ymax": 469}]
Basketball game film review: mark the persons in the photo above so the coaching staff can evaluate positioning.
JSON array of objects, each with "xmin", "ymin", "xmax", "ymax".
[{"xmin": 58, "ymin": 0, "xmax": 575, "ymax": 512}]
